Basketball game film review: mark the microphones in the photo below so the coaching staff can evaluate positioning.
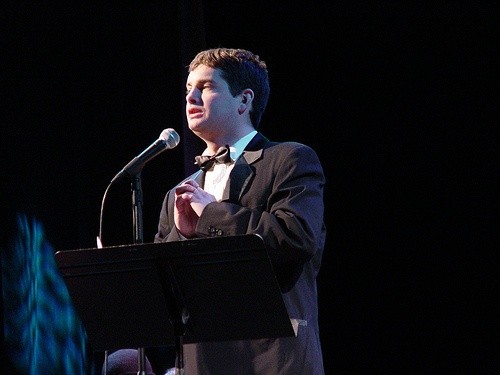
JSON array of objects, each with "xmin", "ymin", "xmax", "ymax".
[{"xmin": 110, "ymin": 127, "xmax": 180, "ymax": 184}]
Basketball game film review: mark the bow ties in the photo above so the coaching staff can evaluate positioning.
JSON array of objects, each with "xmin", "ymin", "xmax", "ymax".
[{"xmin": 195, "ymin": 144, "xmax": 230, "ymax": 173}]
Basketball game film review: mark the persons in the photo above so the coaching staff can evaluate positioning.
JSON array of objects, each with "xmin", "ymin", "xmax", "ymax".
[{"xmin": 154, "ymin": 48, "xmax": 325, "ymax": 375}]
